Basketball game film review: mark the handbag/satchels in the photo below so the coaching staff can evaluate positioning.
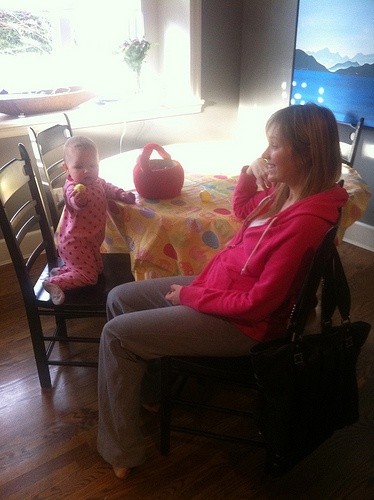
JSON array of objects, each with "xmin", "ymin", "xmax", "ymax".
[{"xmin": 247, "ymin": 179, "xmax": 372, "ymax": 485}]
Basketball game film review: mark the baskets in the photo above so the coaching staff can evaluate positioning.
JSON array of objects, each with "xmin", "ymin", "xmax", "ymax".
[{"xmin": 134, "ymin": 144, "xmax": 184, "ymax": 200}]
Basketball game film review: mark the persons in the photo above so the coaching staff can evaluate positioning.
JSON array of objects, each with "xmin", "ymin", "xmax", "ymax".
[
  {"xmin": 95, "ymin": 103, "xmax": 350, "ymax": 479},
  {"xmin": 43, "ymin": 139, "xmax": 135, "ymax": 307}
]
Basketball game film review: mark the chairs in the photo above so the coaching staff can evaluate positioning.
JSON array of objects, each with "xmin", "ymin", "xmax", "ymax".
[
  {"xmin": 28, "ymin": 113, "xmax": 73, "ymax": 233},
  {"xmin": 0, "ymin": 143, "xmax": 135, "ymax": 393},
  {"xmin": 161, "ymin": 180, "xmax": 345, "ymax": 474},
  {"xmin": 336, "ymin": 118, "xmax": 365, "ymax": 168}
]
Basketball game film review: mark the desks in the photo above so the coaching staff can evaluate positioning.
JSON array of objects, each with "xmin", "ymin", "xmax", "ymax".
[{"xmin": 99, "ymin": 142, "xmax": 374, "ymax": 388}]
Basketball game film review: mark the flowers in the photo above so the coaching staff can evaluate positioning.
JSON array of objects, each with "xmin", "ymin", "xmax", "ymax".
[{"xmin": 116, "ymin": 34, "xmax": 150, "ymax": 86}]
